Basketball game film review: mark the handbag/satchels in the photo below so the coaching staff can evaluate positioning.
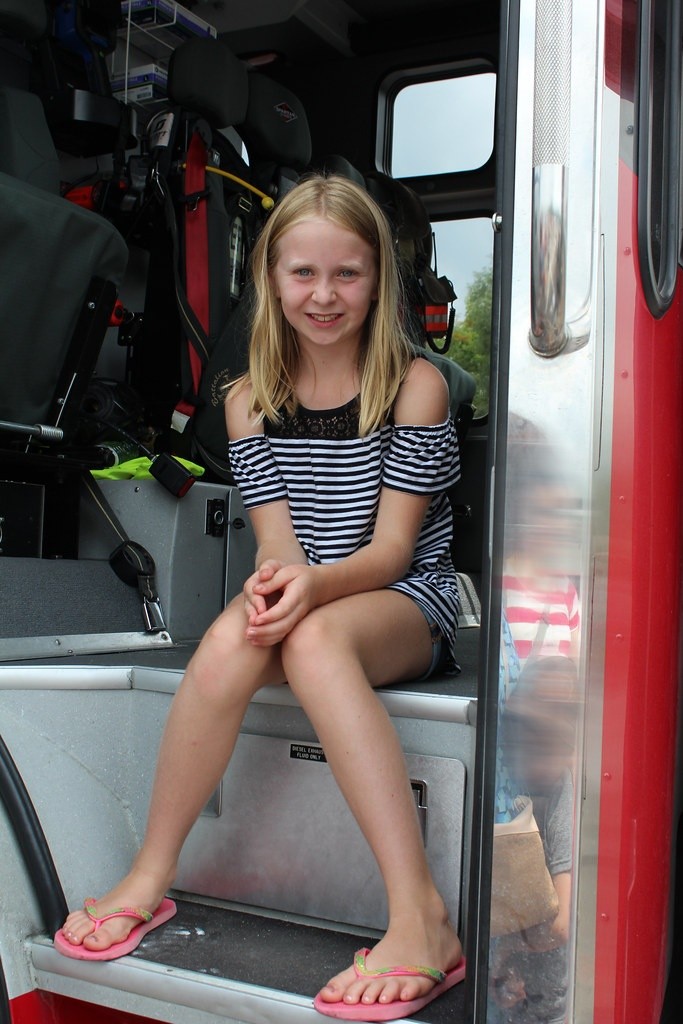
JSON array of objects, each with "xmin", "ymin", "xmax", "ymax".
[
  {"xmin": 504, "ymin": 652, "xmax": 582, "ymax": 789},
  {"xmin": 489, "ymin": 796, "xmax": 560, "ymax": 939}
]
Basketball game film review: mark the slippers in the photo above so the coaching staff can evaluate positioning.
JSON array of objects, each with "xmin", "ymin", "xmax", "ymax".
[
  {"xmin": 314, "ymin": 948, "xmax": 466, "ymax": 1022},
  {"xmin": 55, "ymin": 897, "xmax": 177, "ymax": 961}
]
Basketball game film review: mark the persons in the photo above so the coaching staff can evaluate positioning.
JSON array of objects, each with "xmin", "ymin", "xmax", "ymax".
[
  {"xmin": 496, "ymin": 414, "xmax": 578, "ymax": 1024},
  {"xmin": 53, "ymin": 175, "xmax": 482, "ymax": 1021}
]
